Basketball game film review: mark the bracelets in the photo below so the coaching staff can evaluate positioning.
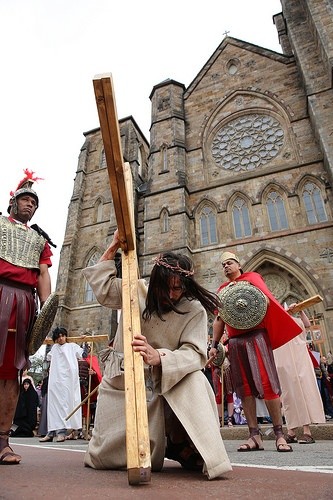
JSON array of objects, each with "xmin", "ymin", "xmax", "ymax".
[{"xmin": 211, "ymin": 340, "xmax": 219, "ymax": 348}]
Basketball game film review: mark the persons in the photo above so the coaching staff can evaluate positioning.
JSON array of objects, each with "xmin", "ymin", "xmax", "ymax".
[
  {"xmin": 82, "ymin": 228, "xmax": 233, "ymax": 480},
  {"xmin": 12, "ymin": 327, "xmax": 333, "ymax": 442},
  {"xmin": 0, "ymin": 169, "xmax": 59, "ymax": 465},
  {"xmin": 207, "ymin": 251, "xmax": 304, "ymax": 452}
]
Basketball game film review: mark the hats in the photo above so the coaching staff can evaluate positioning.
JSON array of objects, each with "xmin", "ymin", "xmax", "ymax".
[{"xmin": 220, "ymin": 251, "xmax": 240, "ymax": 264}]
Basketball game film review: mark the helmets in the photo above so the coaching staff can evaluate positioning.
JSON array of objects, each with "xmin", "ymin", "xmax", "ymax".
[{"xmin": 7, "ymin": 168, "xmax": 45, "ymax": 222}]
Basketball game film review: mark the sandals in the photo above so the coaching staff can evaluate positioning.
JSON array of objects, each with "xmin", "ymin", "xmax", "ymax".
[
  {"xmin": 299, "ymin": 433, "xmax": 315, "ymax": 443},
  {"xmin": 271, "ymin": 425, "xmax": 293, "ymax": 452},
  {"xmin": 286, "ymin": 435, "xmax": 297, "ymax": 443},
  {"xmin": 166, "ymin": 432, "xmax": 205, "ymax": 471},
  {"xmin": 228, "ymin": 417, "xmax": 233, "ymax": 427},
  {"xmin": 0, "ymin": 431, "xmax": 22, "ymax": 464},
  {"xmin": 237, "ymin": 426, "xmax": 265, "ymax": 451},
  {"xmin": 219, "ymin": 417, "xmax": 222, "ymax": 427}
]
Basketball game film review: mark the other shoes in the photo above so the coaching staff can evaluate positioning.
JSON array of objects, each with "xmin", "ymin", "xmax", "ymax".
[
  {"xmin": 83, "ymin": 434, "xmax": 91, "ymax": 440},
  {"xmin": 65, "ymin": 433, "xmax": 77, "ymax": 440},
  {"xmin": 57, "ymin": 434, "xmax": 65, "ymax": 442},
  {"xmin": 35, "ymin": 434, "xmax": 45, "ymax": 436},
  {"xmin": 39, "ymin": 436, "xmax": 53, "ymax": 442}
]
